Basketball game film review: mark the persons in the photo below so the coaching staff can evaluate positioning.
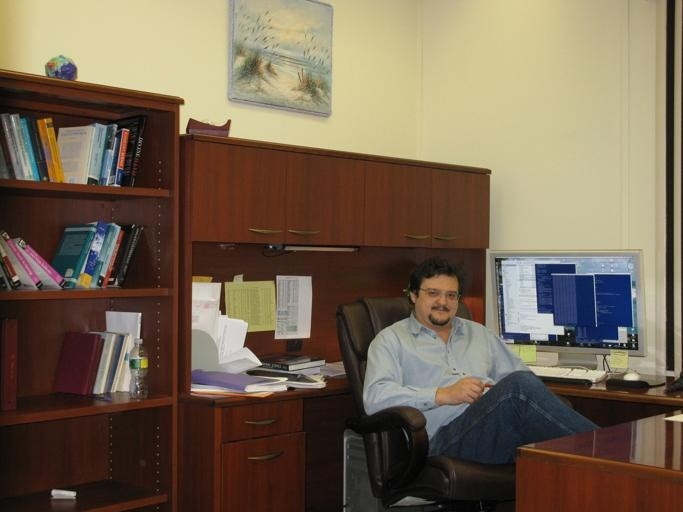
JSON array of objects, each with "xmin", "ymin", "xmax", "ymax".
[{"xmin": 361, "ymin": 256, "xmax": 603, "ymax": 464}]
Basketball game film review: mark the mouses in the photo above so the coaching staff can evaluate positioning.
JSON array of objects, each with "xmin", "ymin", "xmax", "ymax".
[{"xmin": 624, "ymin": 373, "xmax": 641, "ymax": 381}]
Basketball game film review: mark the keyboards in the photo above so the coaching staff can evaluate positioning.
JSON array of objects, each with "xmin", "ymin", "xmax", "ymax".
[{"xmin": 524, "ymin": 365, "xmax": 608, "ymax": 384}]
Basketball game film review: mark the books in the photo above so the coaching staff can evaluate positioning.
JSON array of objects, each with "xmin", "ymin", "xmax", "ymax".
[
  {"xmin": 190, "ymin": 353, "xmax": 328, "ymax": 400},
  {"xmin": 0, "ymin": 221, "xmax": 144, "ymax": 292},
  {"xmin": 1, "ymin": 112, "xmax": 148, "ymax": 187},
  {"xmin": 1, "ymin": 311, "xmax": 142, "ymax": 412}
]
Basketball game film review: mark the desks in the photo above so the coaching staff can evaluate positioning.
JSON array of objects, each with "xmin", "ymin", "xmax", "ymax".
[{"xmin": 180, "ymin": 373, "xmax": 346, "ymax": 512}]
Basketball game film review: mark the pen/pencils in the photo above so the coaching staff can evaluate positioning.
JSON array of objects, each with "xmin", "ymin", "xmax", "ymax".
[{"xmin": 483, "ymin": 384, "xmax": 495, "ymax": 387}]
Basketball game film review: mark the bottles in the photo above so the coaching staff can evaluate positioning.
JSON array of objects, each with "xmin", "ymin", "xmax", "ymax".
[{"xmin": 129, "ymin": 338, "xmax": 149, "ymax": 399}]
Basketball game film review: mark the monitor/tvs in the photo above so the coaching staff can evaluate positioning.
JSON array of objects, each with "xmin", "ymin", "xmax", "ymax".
[{"xmin": 489, "ymin": 250, "xmax": 648, "ymax": 369}]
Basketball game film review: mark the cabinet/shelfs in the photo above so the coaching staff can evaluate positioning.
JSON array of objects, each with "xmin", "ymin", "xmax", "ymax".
[{"xmin": 0, "ymin": 70, "xmax": 185, "ymax": 512}]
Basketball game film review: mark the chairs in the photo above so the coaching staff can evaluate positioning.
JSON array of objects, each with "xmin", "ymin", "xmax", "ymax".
[{"xmin": 337, "ymin": 294, "xmax": 573, "ymax": 511}]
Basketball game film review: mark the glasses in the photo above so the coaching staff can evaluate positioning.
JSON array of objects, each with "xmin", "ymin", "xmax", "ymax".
[{"xmin": 421, "ymin": 287, "xmax": 463, "ymax": 304}]
